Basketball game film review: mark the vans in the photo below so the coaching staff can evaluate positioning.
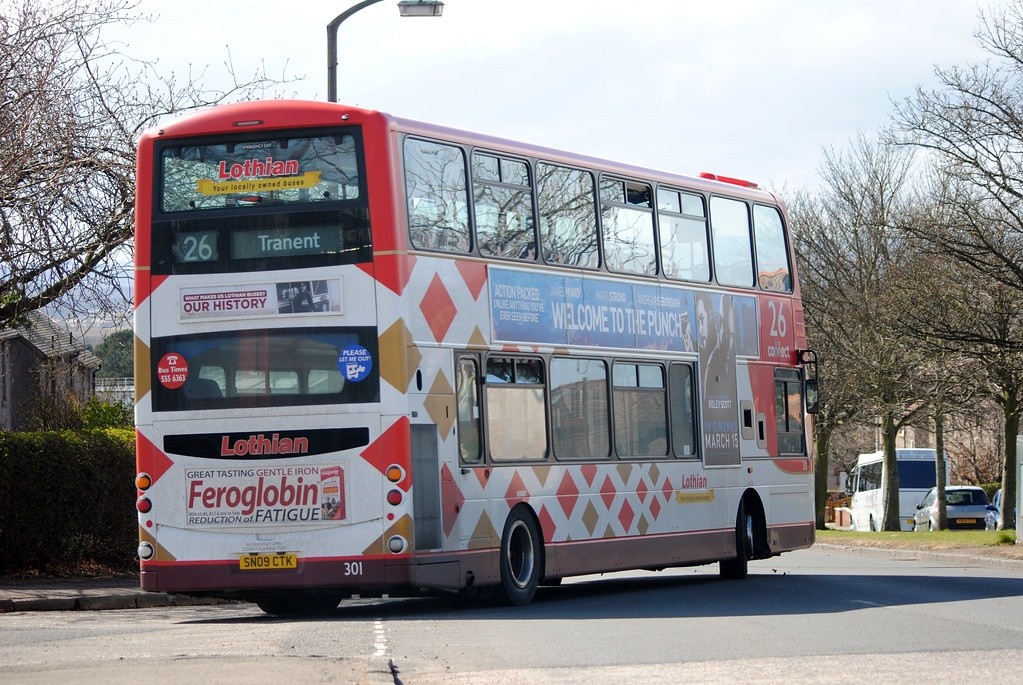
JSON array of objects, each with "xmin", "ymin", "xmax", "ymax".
[{"xmin": 843, "ymin": 449, "xmax": 946, "ymax": 533}]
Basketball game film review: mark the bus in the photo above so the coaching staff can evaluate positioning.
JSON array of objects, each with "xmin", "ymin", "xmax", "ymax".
[{"xmin": 127, "ymin": 100, "xmax": 822, "ymax": 620}]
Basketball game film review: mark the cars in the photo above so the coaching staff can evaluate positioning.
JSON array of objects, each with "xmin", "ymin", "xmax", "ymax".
[
  {"xmin": 985, "ymin": 489, "xmax": 1016, "ymax": 530},
  {"xmin": 913, "ymin": 486, "xmax": 991, "ymax": 532}
]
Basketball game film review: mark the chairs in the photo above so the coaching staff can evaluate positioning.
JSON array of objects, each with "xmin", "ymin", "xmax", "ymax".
[{"xmin": 184, "ymin": 378, "xmax": 222, "ymax": 398}]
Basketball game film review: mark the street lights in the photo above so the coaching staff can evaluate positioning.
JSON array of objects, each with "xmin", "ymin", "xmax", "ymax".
[{"xmin": 325, "ymin": 1, "xmax": 450, "ymax": 104}]
